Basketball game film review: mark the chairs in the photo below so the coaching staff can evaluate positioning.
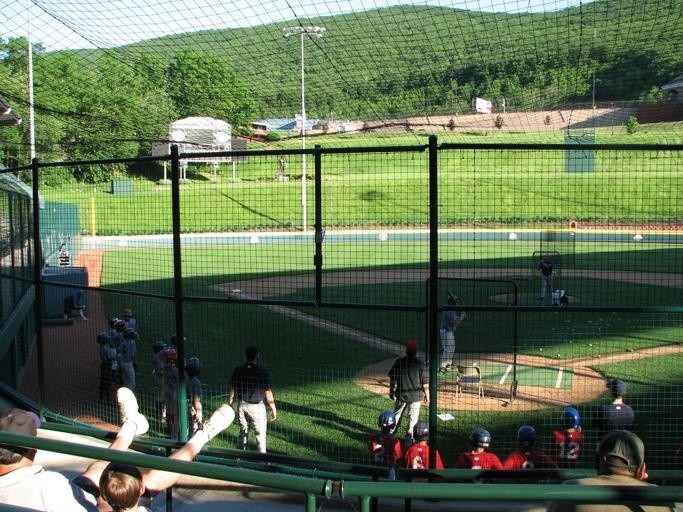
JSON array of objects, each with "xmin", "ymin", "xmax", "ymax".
[{"xmin": 454, "ymin": 365, "xmax": 485, "ymax": 404}]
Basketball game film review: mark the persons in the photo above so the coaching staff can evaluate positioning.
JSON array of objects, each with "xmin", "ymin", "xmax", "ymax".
[
  {"xmin": 439, "ymin": 292, "xmax": 467, "ymax": 373},
  {"xmin": 63, "ymin": 289, "xmax": 88, "ymax": 320},
  {"xmin": 97, "ymin": 308, "xmax": 278, "ymax": 454},
  {"xmin": 59, "ymin": 243, "xmax": 69, "ymax": 266},
  {"xmin": 537, "ymin": 253, "xmax": 555, "ymax": 299},
  {"xmin": 97, "ymin": 462, "xmax": 153, "ymax": 512},
  {"xmin": 0, "ymin": 388, "xmax": 235, "ymax": 512}
]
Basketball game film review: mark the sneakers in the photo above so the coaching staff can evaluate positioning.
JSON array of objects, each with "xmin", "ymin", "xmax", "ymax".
[
  {"xmin": 116, "ymin": 387, "xmax": 150, "ymax": 435},
  {"xmin": 197, "ymin": 403, "xmax": 236, "ymax": 446}
]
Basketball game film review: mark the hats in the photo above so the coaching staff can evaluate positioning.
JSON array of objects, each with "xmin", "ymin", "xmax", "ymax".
[
  {"xmin": 607, "ymin": 379, "xmax": 625, "ymax": 395},
  {"xmin": 405, "ymin": 341, "xmax": 417, "ymax": 352},
  {"xmin": 597, "ymin": 430, "xmax": 645, "ymax": 472},
  {"xmin": 247, "ymin": 345, "xmax": 264, "ymax": 354},
  {"xmin": 0, "ymin": 408, "xmax": 41, "ymax": 436}
]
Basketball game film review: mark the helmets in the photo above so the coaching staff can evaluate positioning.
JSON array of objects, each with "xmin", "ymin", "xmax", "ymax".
[
  {"xmin": 470, "ymin": 428, "xmax": 492, "ymax": 448},
  {"xmin": 152, "ymin": 335, "xmax": 202, "ymax": 375},
  {"xmin": 97, "ymin": 332, "xmax": 110, "ymax": 344},
  {"xmin": 412, "ymin": 422, "xmax": 429, "ymax": 439},
  {"xmin": 560, "ymin": 406, "xmax": 581, "ymax": 429},
  {"xmin": 378, "ymin": 411, "xmax": 396, "ymax": 429},
  {"xmin": 517, "ymin": 425, "xmax": 538, "ymax": 448},
  {"xmin": 110, "ymin": 308, "xmax": 136, "ymax": 339}
]
